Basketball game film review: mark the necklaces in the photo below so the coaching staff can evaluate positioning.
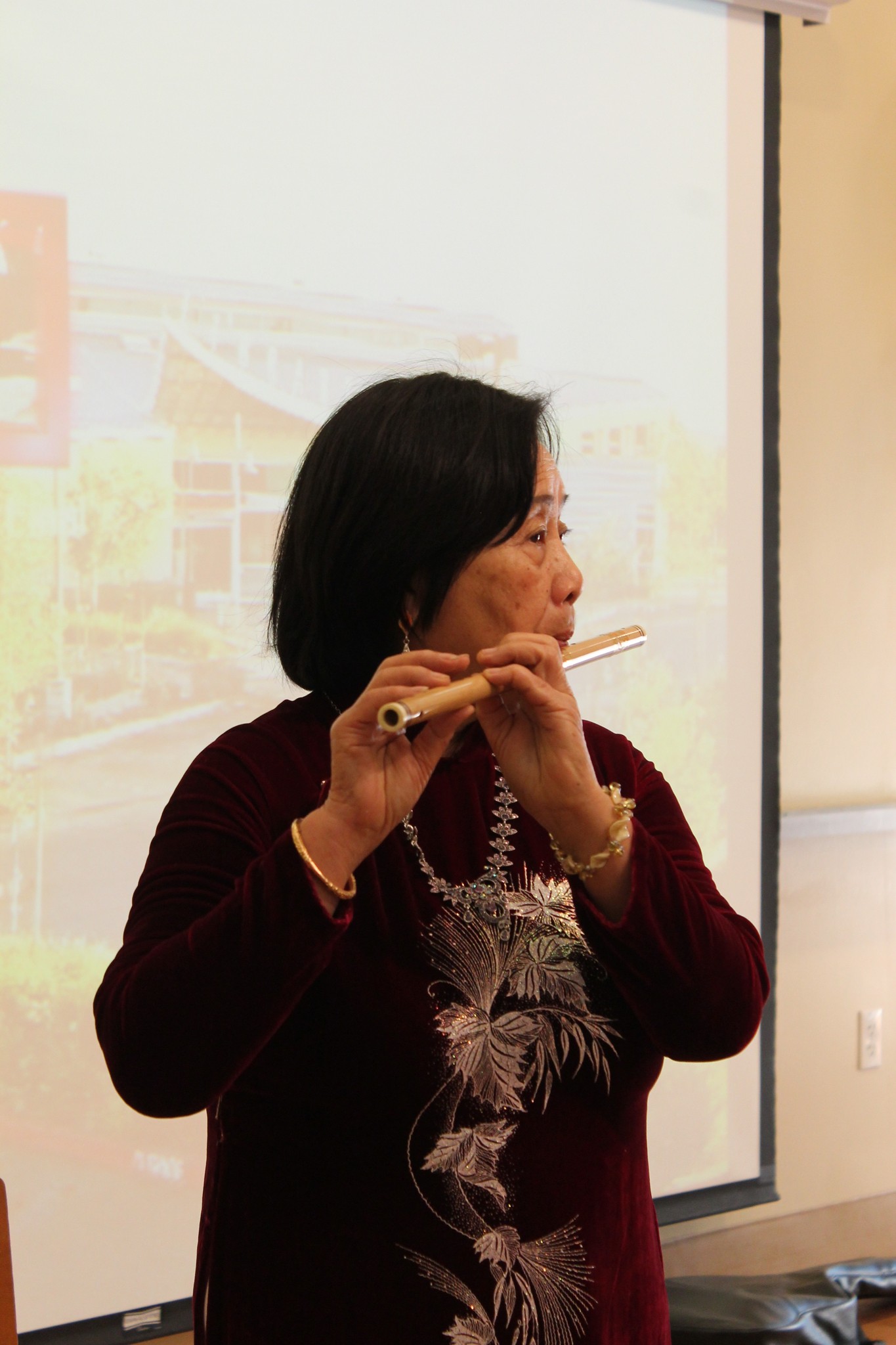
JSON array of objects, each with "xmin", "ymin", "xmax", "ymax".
[{"xmin": 323, "ymin": 690, "xmax": 519, "ymax": 941}]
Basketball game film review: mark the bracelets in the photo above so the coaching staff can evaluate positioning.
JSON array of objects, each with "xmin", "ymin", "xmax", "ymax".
[
  {"xmin": 291, "ymin": 818, "xmax": 357, "ymax": 899},
  {"xmin": 549, "ymin": 782, "xmax": 636, "ymax": 882}
]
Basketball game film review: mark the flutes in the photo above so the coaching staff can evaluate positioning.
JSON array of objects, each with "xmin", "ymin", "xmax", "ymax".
[{"xmin": 376, "ymin": 625, "xmax": 647, "ymax": 737}]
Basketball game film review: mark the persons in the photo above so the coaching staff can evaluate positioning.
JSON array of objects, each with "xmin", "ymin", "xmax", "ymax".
[{"xmin": 93, "ymin": 373, "xmax": 771, "ymax": 1345}]
